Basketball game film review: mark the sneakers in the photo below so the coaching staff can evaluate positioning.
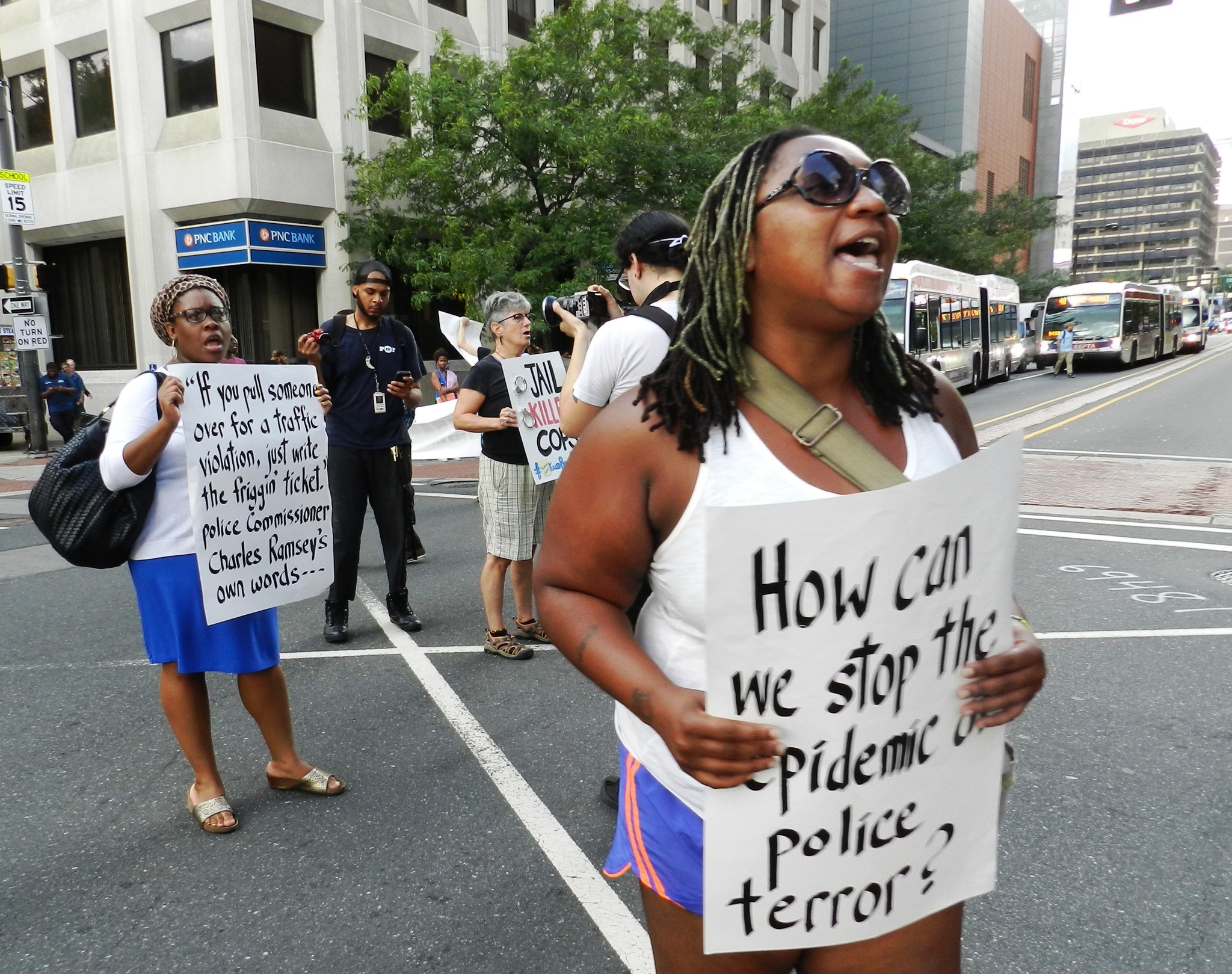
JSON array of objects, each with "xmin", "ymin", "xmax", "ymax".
[
  {"xmin": 386, "ymin": 589, "xmax": 422, "ymax": 632},
  {"xmin": 485, "ymin": 628, "xmax": 535, "ymax": 660},
  {"xmin": 1054, "ymin": 373, "xmax": 1059, "ymax": 379},
  {"xmin": 384, "ymin": 548, "xmax": 427, "ymax": 565},
  {"xmin": 1068, "ymin": 373, "xmax": 1076, "ymax": 378},
  {"xmin": 601, "ymin": 774, "xmax": 621, "ymax": 810},
  {"xmin": 515, "ymin": 615, "xmax": 550, "ymax": 644},
  {"xmin": 323, "ymin": 598, "xmax": 350, "ymax": 643}
]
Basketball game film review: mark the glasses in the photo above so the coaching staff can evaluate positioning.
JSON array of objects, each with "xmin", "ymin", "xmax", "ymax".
[
  {"xmin": 169, "ymin": 306, "xmax": 232, "ymax": 323},
  {"xmin": 752, "ymin": 148, "xmax": 913, "ymax": 219},
  {"xmin": 614, "ymin": 250, "xmax": 632, "ymax": 291},
  {"xmin": 498, "ymin": 312, "xmax": 536, "ymax": 324}
]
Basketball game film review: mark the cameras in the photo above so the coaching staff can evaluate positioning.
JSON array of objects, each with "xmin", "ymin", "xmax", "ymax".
[
  {"xmin": 541, "ymin": 286, "xmax": 610, "ymax": 330},
  {"xmin": 304, "ymin": 328, "xmax": 332, "ymax": 348}
]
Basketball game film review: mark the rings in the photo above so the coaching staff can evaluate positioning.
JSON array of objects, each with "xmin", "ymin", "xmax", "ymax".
[{"xmin": 503, "ymin": 417, "xmax": 507, "ymax": 423}]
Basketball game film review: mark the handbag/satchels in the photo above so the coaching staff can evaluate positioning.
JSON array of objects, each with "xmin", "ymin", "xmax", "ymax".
[{"xmin": 27, "ymin": 369, "xmax": 180, "ymax": 571}]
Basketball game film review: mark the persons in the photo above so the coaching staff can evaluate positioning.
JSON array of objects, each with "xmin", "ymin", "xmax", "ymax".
[
  {"xmin": 431, "ymin": 349, "xmax": 459, "ymax": 404},
  {"xmin": 66, "ymin": 359, "xmax": 92, "ymax": 400},
  {"xmin": 39, "ymin": 363, "xmax": 76, "ymax": 442},
  {"xmin": 299, "ymin": 261, "xmax": 426, "ymax": 642},
  {"xmin": 1208, "ymin": 316, "xmax": 1218, "ymax": 334},
  {"xmin": 534, "ymin": 121, "xmax": 1045, "ymax": 970},
  {"xmin": 218, "ymin": 334, "xmax": 244, "ymax": 363},
  {"xmin": 1054, "ymin": 322, "xmax": 1075, "ymax": 379},
  {"xmin": 551, "ymin": 209, "xmax": 689, "ymax": 809},
  {"xmin": 458, "ymin": 315, "xmax": 494, "ymax": 361},
  {"xmin": 271, "ymin": 349, "xmax": 288, "ymax": 365},
  {"xmin": 60, "ymin": 363, "xmax": 85, "ymax": 417},
  {"xmin": 98, "ymin": 274, "xmax": 346, "ymax": 834},
  {"xmin": 453, "ymin": 291, "xmax": 551, "ymax": 659},
  {"xmin": 336, "ymin": 309, "xmax": 426, "ymax": 563}
]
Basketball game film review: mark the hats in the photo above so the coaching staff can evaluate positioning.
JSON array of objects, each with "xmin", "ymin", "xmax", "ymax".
[
  {"xmin": 270, "ymin": 349, "xmax": 283, "ymax": 361},
  {"xmin": 352, "ymin": 260, "xmax": 392, "ymax": 299},
  {"xmin": 150, "ymin": 274, "xmax": 231, "ymax": 347}
]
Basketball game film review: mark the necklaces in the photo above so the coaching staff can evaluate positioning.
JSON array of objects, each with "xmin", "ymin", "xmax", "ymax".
[{"xmin": 495, "ymin": 351, "xmax": 505, "ymax": 359}]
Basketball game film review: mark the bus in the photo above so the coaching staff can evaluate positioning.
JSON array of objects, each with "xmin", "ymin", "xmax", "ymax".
[
  {"xmin": 1030, "ymin": 281, "xmax": 1185, "ymax": 367},
  {"xmin": 881, "ymin": 260, "xmax": 1021, "ymax": 396},
  {"xmin": 1181, "ymin": 287, "xmax": 1208, "ymax": 353}
]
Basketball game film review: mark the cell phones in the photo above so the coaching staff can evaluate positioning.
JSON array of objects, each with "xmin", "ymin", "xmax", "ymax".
[{"xmin": 393, "ymin": 370, "xmax": 411, "ymax": 385}]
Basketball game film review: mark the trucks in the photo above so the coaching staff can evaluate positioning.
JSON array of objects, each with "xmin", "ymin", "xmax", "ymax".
[{"xmin": 1018, "ymin": 300, "xmax": 1046, "ymax": 371}]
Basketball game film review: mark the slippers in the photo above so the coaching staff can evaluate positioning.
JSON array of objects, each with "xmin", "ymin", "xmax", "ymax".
[
  {"xmin": 266, "ymin": 763, "xmax": 348, "ymax": 798},
  {"xmin": 185, "ymin": 786, "xmax": 238, "ymax": 834}
]
